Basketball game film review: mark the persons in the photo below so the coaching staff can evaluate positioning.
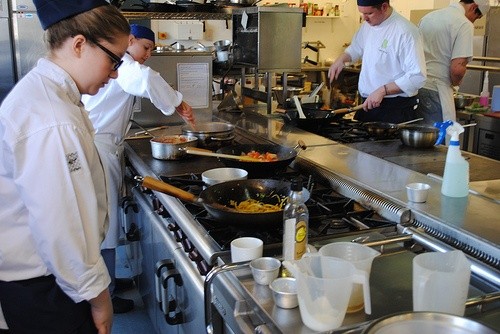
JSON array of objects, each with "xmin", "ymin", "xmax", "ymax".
[
  {"xmin": 417, "ymin": 0, "xmax": 490, "ymax": 146},
  {"xmin": 328, "ymin": 0, "xmax": 427, "ymax": 125},
  {"xmin": 0, "ymin": 0, "xmax": 131, "ymax": 334},
  {"xmin": 80, "ymin": 23, "xmax": 195, "ymax": 314}
]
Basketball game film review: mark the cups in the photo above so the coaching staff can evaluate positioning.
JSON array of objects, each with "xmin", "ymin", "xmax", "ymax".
[
  {"xmin": 250, "ymin": 257, "xmax": 283, "ymax": 285},
  {"xmin": 405, "ymin": 181, "xmax": 431, "ymax": 203},
  {"xmin": 230, "ymin": 236, "xmax": 264, "ymax": 265},
  {"xmin": 269, "ymin": 277, "xmax": 302, "ymax": 309}
]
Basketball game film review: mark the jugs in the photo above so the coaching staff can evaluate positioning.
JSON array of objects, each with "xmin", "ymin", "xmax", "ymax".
[{"xmin": 287, "ymin": 242, "xmax": 478, "ymax": 331}]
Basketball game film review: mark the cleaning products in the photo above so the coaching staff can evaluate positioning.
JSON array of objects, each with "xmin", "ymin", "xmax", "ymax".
[{"xmin": 432, "ymin": 119, "xmax": 469, "ymax": 197}]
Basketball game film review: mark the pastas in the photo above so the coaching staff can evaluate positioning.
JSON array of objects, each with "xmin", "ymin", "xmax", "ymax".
[{"xmin": 234, "ymin": 195, "xmax": 285, "ymax": 212}]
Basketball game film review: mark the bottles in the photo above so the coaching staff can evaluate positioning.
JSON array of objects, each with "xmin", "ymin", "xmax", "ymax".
[
  {"xmin": 281, "ymin": 175, "xmax": 308, "ymax": 269},
  {"xmin": 294, "ymin": 0, "xmax": 341, "ymax": 16}
]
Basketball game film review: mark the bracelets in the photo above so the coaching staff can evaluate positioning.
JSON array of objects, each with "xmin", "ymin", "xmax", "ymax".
[{"xmin": 385, "ymin": 84, "xmax": 387, "ymax": 97}]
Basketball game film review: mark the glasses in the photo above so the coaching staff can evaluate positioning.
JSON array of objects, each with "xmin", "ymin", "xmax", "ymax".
[{"xmin": 72, "ymin": 32, "xmax": 123, "ymax": 72}]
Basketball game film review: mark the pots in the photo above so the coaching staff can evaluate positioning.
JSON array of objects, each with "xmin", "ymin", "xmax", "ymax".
[
  {"xmin": 139, "ymin": 175, "xmax": 310, "ymax": 228},
  {"xmin": 205, "ymin": 133, "xmax": 307, "ymax": 178},
  {"xmin": 122, "ymin": 0, "xmax": 253, "ymax": 13},
  {"xmin": 129, "ymin": 118, "xmax": 199, "ymax": 160},
  {"xmin": 181, "ymin": 119, "xmax": 236, "ymax": 147}
]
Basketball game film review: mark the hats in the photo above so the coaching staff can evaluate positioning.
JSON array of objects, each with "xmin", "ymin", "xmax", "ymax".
[
  {"xmin": 33, "ymin": 0, "xmax": 109, "ymax": 31},
  {"xmin": 473, "ymin": 0, "xmax": 489, "ymax": 15},
  {"xmin": 357, "ymin": 0, "xmax": 389, "ymax": 6},
  {"xmin": 130, "ymin": 24, "xmax": 156, "ymax": 42}
]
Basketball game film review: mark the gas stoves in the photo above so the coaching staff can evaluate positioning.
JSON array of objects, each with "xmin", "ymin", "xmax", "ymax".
[
  {"xmin": 139, "ymin": 153, "xmax": 403, "ymax": 276},
  {"xmin": 276, "ymin": 106, "xmax": 401, "ymax": 144}
]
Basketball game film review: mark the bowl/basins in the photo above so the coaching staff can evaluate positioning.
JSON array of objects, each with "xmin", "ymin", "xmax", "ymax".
[
  {"xmin": 397, "ymin": 126, "xmax": 440, "ymax": 148},
  {"xmin": 453, "ymin": 94, "xmax": 476, "ymax": 109},
  {"xmin": 365, "ymin": 117, "xmax": 425, "ymax": 139},
  {"xmin": 201, "ymin": 168, "xmax": 249, "ymax": 191},
  {"xmin": 282, "ymin": 99, "xmax": 367, "ymax": 126},
  {"xmin": 361, "ymin": 311, "xmax": 500, "ymax": 334}
]
